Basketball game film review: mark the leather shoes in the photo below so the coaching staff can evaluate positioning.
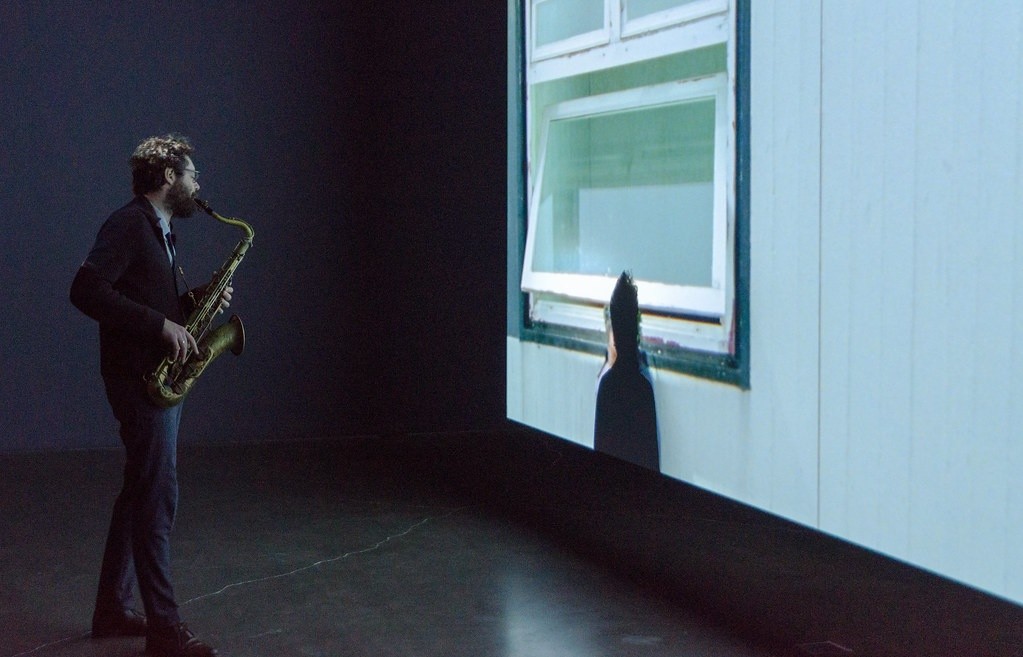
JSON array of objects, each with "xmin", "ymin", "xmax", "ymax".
[
  {"xmin": 92, "ymin": 608, "xmax": 149, "ymax": 638},
  {"xmin": 147, "ymin": 622, "xmax": 219, "ymax": 657}
]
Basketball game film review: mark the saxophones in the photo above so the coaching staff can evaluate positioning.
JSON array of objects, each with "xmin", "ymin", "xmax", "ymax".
[{"xmin": 141, "ymin": 195, "xmax": 256, "ymax": 410}]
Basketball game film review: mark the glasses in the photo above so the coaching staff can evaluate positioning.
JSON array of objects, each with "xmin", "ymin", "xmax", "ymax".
[{"xmin": 177, "ymin": 167, "xmax": 200, "ymax": 182}]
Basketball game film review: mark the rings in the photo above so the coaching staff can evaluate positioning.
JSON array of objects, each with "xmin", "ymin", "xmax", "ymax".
[{"xmin": 180, "ymin": 341, "xmax": 185, "ymax": 345}]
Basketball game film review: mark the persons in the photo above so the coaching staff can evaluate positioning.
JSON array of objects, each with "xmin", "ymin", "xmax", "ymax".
[{"xmin": 68, "ymin": 136, "xmax": 233, "ymax": 656}]
